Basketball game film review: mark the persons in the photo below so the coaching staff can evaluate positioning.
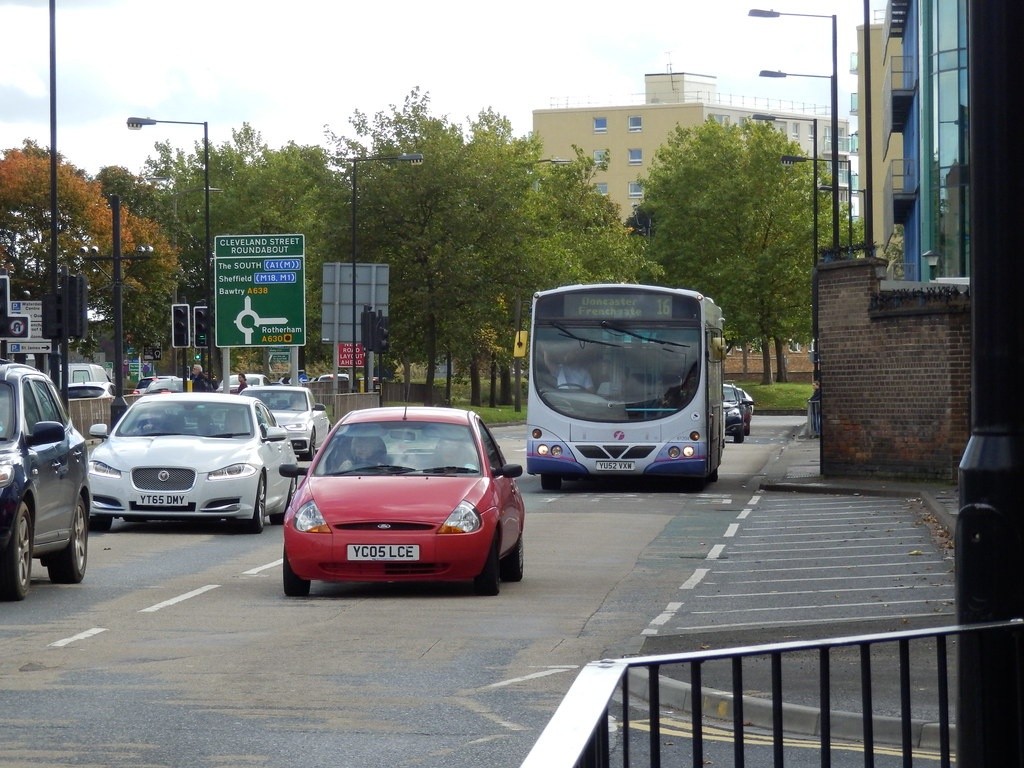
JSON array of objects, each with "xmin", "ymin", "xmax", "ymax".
[
  {"xmin": 193, "ymin": 364, "xmax": 213, "ymax": 392},
  {"xmin": 238, "ymin": 373, "xmax": 247, "ymax": 395},
  {"xmin": 539, "ymin": 341, "xmax": 595, "ymax": 394},
  {"xmin": 806, "ymin": 381, "xmax": 820, "ymax": 439}
]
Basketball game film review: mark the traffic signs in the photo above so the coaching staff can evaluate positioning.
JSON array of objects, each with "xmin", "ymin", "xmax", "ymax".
[{"xmin": 214, "ymin": 234, "xmax": 307, "ymax": 348}]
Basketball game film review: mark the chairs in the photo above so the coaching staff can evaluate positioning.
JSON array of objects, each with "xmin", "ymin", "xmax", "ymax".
[
  {"xmin": 289, "ymin": 394, "xmax": 306, "ymax": 411},
  {"xmin": 225, "ymin": 410, "xmax": 246, "ymax": 437},
  {"xmin": 162, "ymin": 414, "xmax": 185, "ymax": 434},
  {"xmin": 342, "ymin": 433, "xmax": 395, "ymax": 470}
]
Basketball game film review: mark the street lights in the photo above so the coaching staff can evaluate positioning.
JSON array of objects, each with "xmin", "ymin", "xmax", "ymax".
[
  {"xmin": 127, "ymin": 117, "xmax": 224, "ymax": 391},
  {"xmin": 759, "ymin": 70, "xmax": 833, "ymax": 101},
  {"xmin": 781, "ymin": 153, "xmax": 853, "ymax": 251},
  {"xmin": 351, "ymin": 154, "xmax": 424, "ymax": 393},
  {"xmin": 749, "ymin": 9, "xmax": 839, "ymax": 249},
  {"xmin": 820, "ymin": 185, "xmax": 868, "ymax": 257},
  {"xmin": 751, "ymin": 113, "xmax": 818, "ymax": 267}
]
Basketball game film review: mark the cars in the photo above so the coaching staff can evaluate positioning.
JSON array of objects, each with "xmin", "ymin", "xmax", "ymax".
[
  {"xmin": 723, "ymin": 383, "xmax": 754, "ymax": 443},
  {"xmin": 48, "ymin": 364, "xmax": 189, "ymax": 398},
  {"xmin": 237, "ymin": 388, "xmax": 331, "ymax": 462},
  {"xmin": 88, "ymin": 392, "xmax": 299, "ymax": 535},
  {"xmin": 215, "ymin": 373, "xmax": 349, "ymax": 393},
  {"xmin": 278, "ymin": 406, "xmax": 526, "ymax": 597}
]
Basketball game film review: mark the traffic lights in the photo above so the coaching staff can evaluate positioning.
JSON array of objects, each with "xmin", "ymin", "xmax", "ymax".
[
  {"xmin": 193, "ymin": 306, "xmax": 208, "ymax": 348},
  {"xmin": 171, "ymin": 304, "xmax": 191, "ymax": 348},
  {"xmin": 0, "ymin": 275, "xmax": 30, "ymax": 340},
  {"xmin": 194, "ymin": 348, "xmax": 201, "ymax": 359}
]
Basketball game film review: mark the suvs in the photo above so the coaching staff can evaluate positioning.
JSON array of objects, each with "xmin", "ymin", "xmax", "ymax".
[{"xmin": 0, "ymin": 363, "xmax": 93, "ymax": 602}]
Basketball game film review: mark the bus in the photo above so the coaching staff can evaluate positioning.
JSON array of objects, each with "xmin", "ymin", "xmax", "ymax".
[{"xmin": 514, "ymin": 283, "xmax": 729, "ymax": 492}]
